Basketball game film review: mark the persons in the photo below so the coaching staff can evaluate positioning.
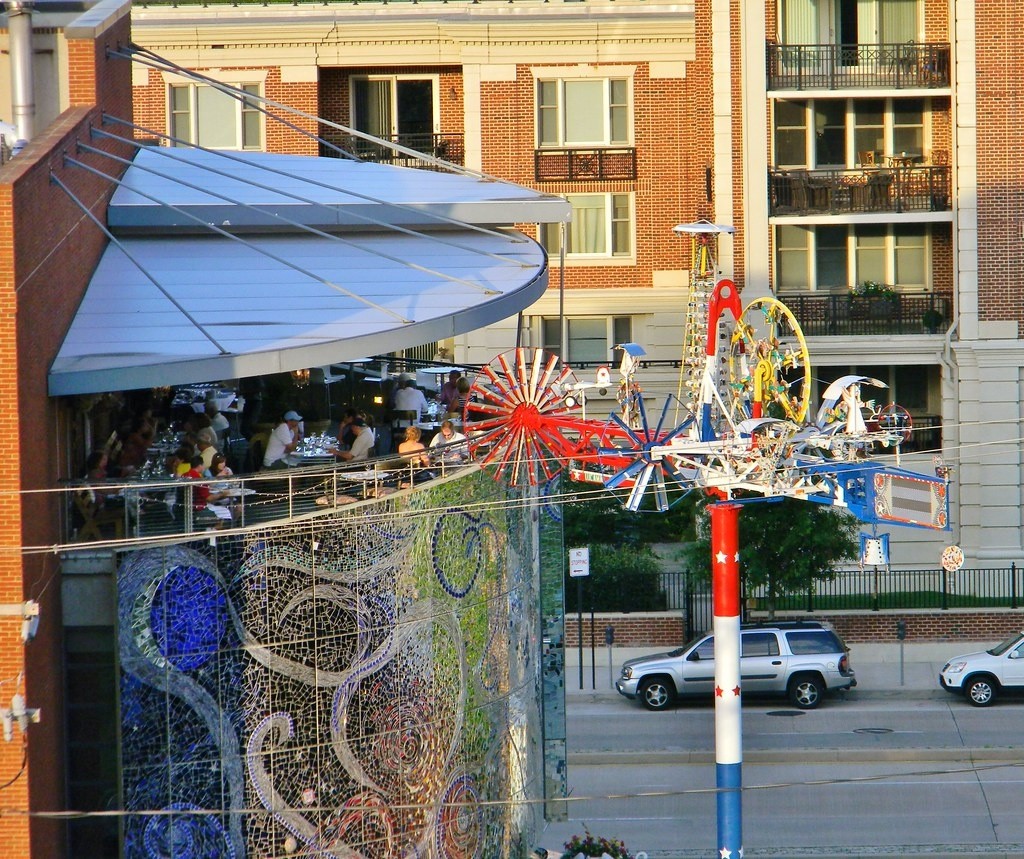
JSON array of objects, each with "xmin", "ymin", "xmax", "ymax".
[
  {"xmin": 399, "ymin": 427, "xmax": 436, "ymax": 485},
  {"xmin": 434, "ymin": 371, "xmax": 475, "ymax": 422},
  {"xmin": 263, "ymin": 411, "xmax": 302, "ymax": 497},
  {"xmin": 80, "ymin": 409, "xmax": 158, "ymax": 535},
  {"xmin": 172, "ymin": 401, "xmax": 241, "ymax": 530},
  {"xmin": 368, "ymin": 374, "xmax": 429, "ymax": 442},
  {"xmin": 318, "ymin": 410, "xmax": 375, "ymax": 498},
  {"xmin": 846, "ymin": 386, "xmax": 867, "ymax": 435},
  {"xmin": 427, "ymin": 421, "xmax": 469, "ymax": 477}
]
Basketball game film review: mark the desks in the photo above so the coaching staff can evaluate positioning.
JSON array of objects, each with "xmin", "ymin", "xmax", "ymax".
[
  {"xmin": 415, "ymin": 421, "xmax": 478, "ymax": 444},
  {"xmin": 373, "ymin": 143, "xmax": 412, "ymax": 165},
  {"xmin": 879, "ymin": 153, "xmax": 922, "ymax": 211},
  {"xmin": 289, "ymin": 436, "xmax": 341, "ymax": 493},
  {"xmin": 170, "ymin": 387, "xmax": 244, "ymax": 442},
  {"xmin": 420, "ymin": 367, "xmax": 468, "ymax": 389},
  {"xmin": 905, "ymin": 47, "xmax": 945, "ymax": 87},
  {"xmin": 339, "ymin": 358, "xmax": 374, "ymax": 381},
  {"xmin": 142, "ymin": 432, "xmax": 182, "ymax": 476},
  {"xmin": 420, "ymin": 405, "xmax": 447, "ymax": 421},
  {"xmin": 105, "ymin": 476, "xmax": 193, "ymax": 521}
]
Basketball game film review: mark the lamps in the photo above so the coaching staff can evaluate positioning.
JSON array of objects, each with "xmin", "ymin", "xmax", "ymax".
[{"xmin": 448, "ymin": 87, "xmax": 456, "ymax": 100}]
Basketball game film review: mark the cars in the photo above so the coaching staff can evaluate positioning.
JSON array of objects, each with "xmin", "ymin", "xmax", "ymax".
[{"xmin": 938, "ymin": 632, "xmax": 1024, "ymax": 705}]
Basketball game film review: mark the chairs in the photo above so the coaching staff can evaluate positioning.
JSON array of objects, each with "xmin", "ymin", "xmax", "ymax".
[
  {"xmin": 344, "ymin": 134, "xmax": 448, "ymax": 163},
  {"xmin": 67, "ymin": 367, "xmax": 466, "ymax": 543},
  {"xmin": 769, "ymin": 150, "xmax": 952, "ymax": 213},
  {"xmin": 826, "ymin": 286, "xmax": 902, "ymax": 335}
]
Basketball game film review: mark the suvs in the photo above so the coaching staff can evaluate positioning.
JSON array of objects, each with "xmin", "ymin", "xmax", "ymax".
[{"xmin": 616, "ymin": 618, "xmax": 858, "ymax": 710}]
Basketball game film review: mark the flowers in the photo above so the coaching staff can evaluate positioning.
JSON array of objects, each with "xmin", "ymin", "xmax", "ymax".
[
  {"xmin": 848, "ymin": 280, "xmax": 896, "ymax": 302},
  {"xmin": 561, "ymin": 822, "xmax": 639, "ymax": 859}
]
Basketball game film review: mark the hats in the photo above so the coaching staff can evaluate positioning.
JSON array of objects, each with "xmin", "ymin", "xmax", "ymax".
[
  {"xmin": 346, "ymin": 418, "xmax": 366, "ymax": 427},
  {"xmin": 284, "ymin": 411, "xmax": 302, "ymax": 421}
]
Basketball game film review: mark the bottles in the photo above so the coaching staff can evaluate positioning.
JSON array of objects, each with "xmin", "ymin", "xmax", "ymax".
[
  {"xmin": 309, "ymin": 430, "xmax": 327, "ymax": 444},
  {"xmin": 438, "ymin": 406, "xmax": 446, "ymax": 425}
]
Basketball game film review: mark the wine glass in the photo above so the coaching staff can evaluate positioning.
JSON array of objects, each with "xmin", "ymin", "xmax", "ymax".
[{"xmin": 431, "ymin": 414, "xmax": 436, "ymax": 427}]
